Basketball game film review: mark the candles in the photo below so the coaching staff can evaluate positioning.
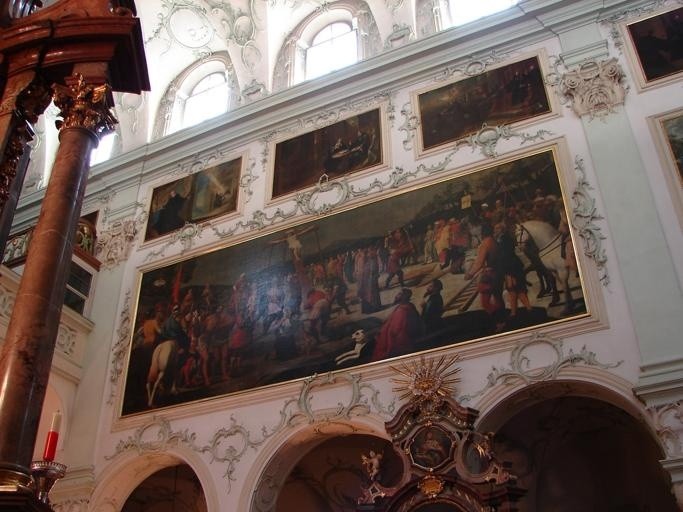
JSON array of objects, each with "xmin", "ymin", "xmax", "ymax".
[{"xmin": 43, "ymin": 409, "xmax": 62, "ymax": 462}]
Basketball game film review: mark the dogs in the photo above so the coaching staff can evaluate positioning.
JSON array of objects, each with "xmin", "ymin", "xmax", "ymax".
[{"xmin": 334, "ymin": 328, "xmax": 369, "ymax": 366}]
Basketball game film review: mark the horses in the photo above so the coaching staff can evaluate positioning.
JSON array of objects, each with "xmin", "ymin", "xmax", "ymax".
[
  {"xmin": 146, "ymin": 340, "xmax": 177, "ymax": 406},
  {"xmin": 513, "ymin": 220, "xmax": 579, "ymax": 316}
]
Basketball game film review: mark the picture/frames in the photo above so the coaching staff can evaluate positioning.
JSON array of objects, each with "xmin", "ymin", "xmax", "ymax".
[
  {"xmin": 138, "ymin": 147, "xmax": 249, "ymax": 250},
  {"xmin": 408, "ymin": 48, "xmax": 563, "ymax": 166},
  {"xmin": 264, "ymin": 99, "xmax": 393, "ymax": 211},
  {"xmin": 105, "ymin": 136, "xmax": 610, "ymax": 432},
  {"xmin": 644, "ymin": 106, "xmax": 682, "ymax": 228},
  {"xmin": 619, "ymin": 0, "xmax": 682, "ymax": 94}
]
